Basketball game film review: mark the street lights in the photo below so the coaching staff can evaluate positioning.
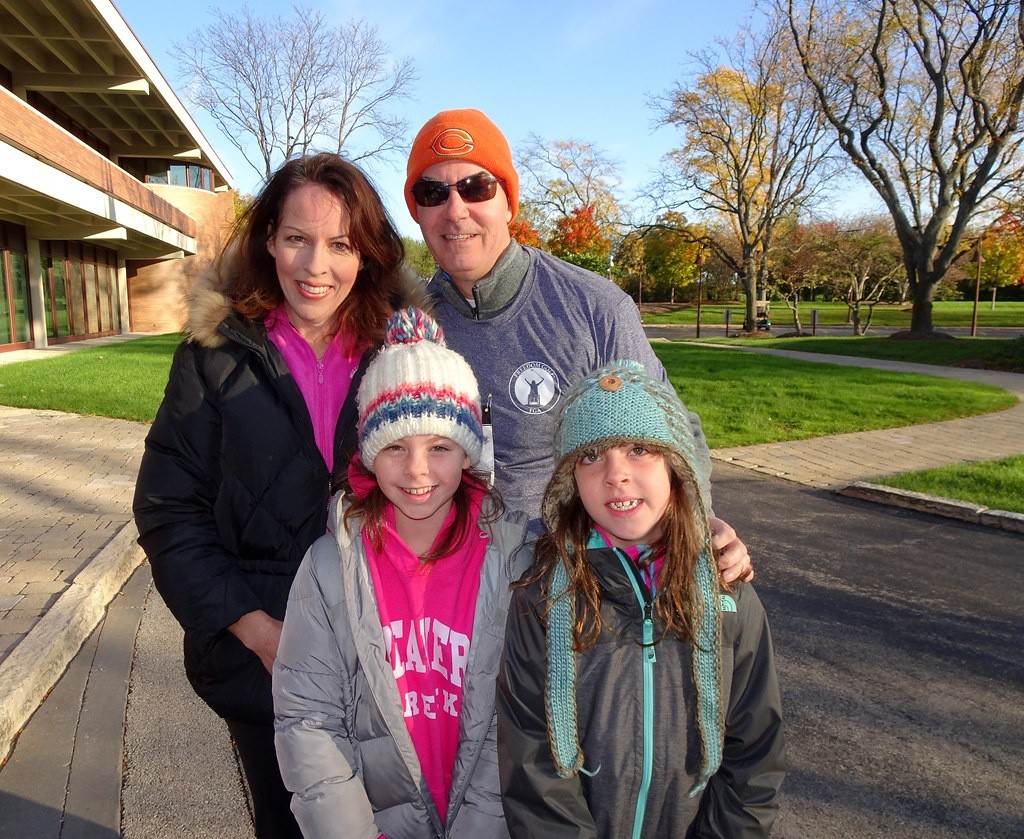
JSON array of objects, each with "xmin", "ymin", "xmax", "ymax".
[
  {"xmin": 692, "ymin": 248, "xmax": 706, "ymax": 339},
  {"xmin": 637, "ymin": 256, "xmax": 645, "ymax": 313},
  {"xmin": 969, "ymin": 235, "xmax": 987, "ymax": 337}
]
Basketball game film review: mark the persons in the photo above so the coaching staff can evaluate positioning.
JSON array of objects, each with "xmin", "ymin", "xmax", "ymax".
[
  {"xmin": 494, "ymin": 355, "xmax": 792, "ymax": 839},
  {"xmin": 130, "ymin": 153, "xmax": 441, "ymax": 839},
  {"xmin": 402, "ymin": 106, "xmax": 760, "ymax": 586},
  {"xmin": 272, "ymin": 304, "xmax": 542, "ymax": 839}
]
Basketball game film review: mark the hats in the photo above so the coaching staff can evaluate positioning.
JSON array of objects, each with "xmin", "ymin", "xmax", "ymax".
[
  {"xmin": 544, "ymin": 357, "xmax": 726, "ymax": 797},
  {"xmin": 404, "ymin": 108, "xmax": 519, "ymax": 226},
  {"xmin": 356, "ymin": 305, "xmax": 482, "ymax": 473}
]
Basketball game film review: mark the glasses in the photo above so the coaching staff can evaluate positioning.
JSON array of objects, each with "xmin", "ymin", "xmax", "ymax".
[{"xmin": 411, "ymin": 172, "xmax": 503, "ymax": 208}]
